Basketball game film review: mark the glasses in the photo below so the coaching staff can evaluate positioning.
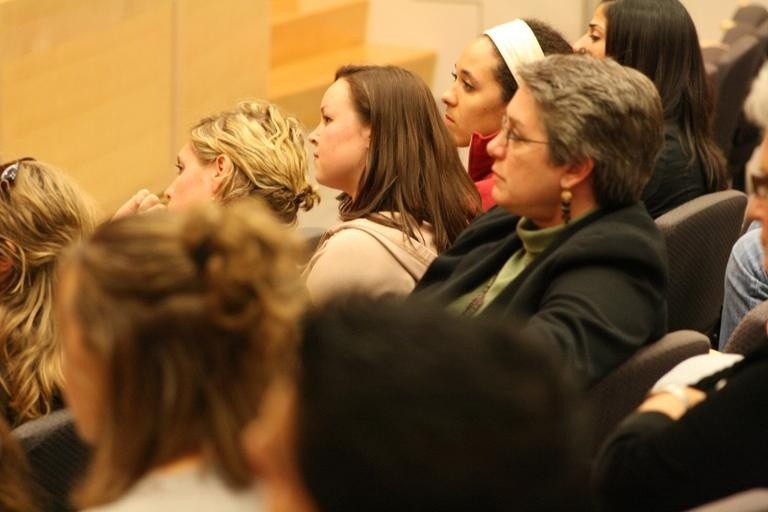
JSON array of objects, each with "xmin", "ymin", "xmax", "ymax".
[
  {"xmin": 500, "ymin": 114, "xmax": 559, "ymax": 149},
  {"xmin": 0, "ymin": 155, "xmax": 38, "ymax": 205}
]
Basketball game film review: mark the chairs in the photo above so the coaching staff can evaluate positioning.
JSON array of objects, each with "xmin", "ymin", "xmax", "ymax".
[
  {"xmin": 6, "ymin": 404, "xmax": 100, "ymax": 511},
  {"xmin": 686, "ymin": 0, "xmax": 768, "ymax": 190},
  {"xmin": 721, "ymin": 297, "xmax": 768, "ymax": 360},
  {"xmin": 654, "ymin": 185, "xmax": 745, "ymax": 347},
  {"xmin": 582, "ymin": 325, "xmax": 708, "ymax": 440}
]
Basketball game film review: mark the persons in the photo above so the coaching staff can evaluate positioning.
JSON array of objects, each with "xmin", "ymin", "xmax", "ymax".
[
  {"xmin": 569, "ymin": 1, "xmax": 730, "ymax": 221},
  {"xmin": 717, "ymin": 57, "xmax": 766, "ymax": 353},
  {"xmin": 297, "ymin": 61, "xmax": 479, "ymax": 317},
  {"xmin": 436, "ymin": 17, "xmax": 575, "ymax": 220},
  {"xmin": 0, "ymin": 157, "xmax": 95, "ymax": 422},
  {"xmin": 601, "ymin": 351, "xmax": 768, "ymax": 512},
  {"xmin": 50, "ymin": 192, "xmax": 301, "ymax": 510},
  {"xmin": 237, "ymin": 286, "xmax": 597, "ymax": 511},
  {"xmin": 104, "ymin": 99, "xmax": 319, "ymax": 244}
]
